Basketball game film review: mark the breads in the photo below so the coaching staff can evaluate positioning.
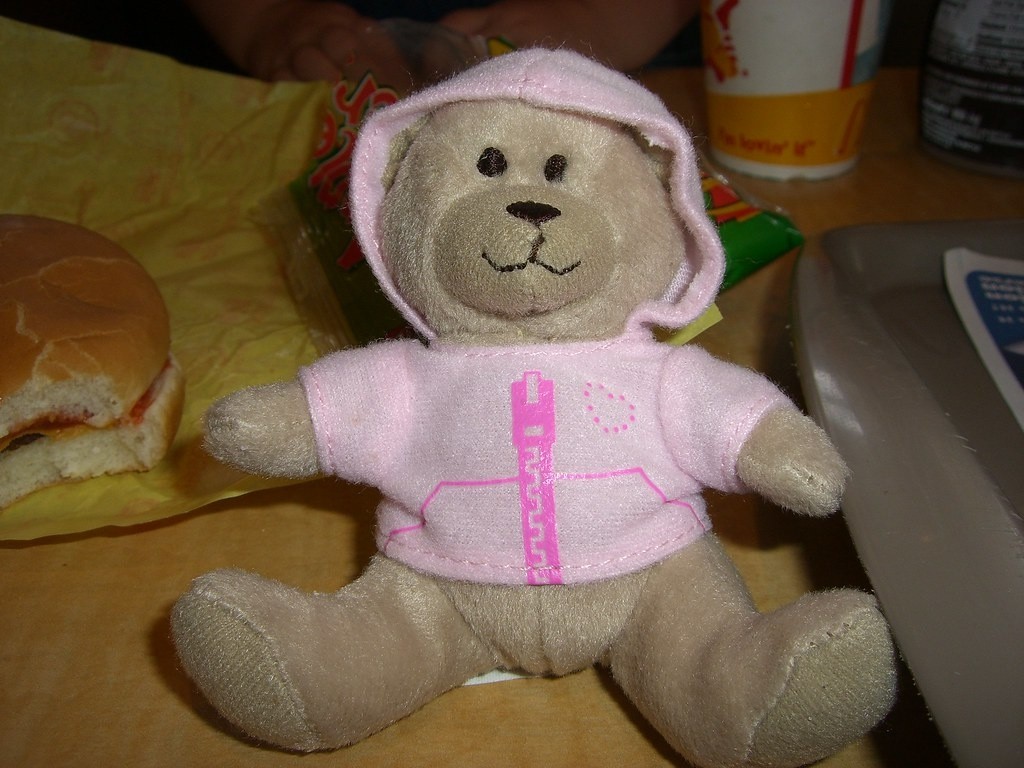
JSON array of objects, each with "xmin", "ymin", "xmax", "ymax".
[{"xmin": 0, "ymin": 214, "xmax": 183, "ymax": 507}]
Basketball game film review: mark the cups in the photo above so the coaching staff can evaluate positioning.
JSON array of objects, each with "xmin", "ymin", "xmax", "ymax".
[
  {"xmin": 915, "ymin": 0, "xmax": 1024, "ymax": 179},
  {"xmin": 701, "ymin": 0, "xmax": 885, "ymax": 187}
]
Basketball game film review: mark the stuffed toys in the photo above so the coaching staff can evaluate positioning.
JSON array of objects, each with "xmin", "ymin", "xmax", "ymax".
[{"xmin": 171, "ymin": 45, "xmax": 896, "ymax": 767}]
